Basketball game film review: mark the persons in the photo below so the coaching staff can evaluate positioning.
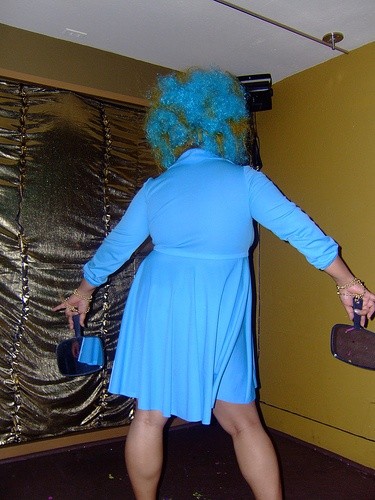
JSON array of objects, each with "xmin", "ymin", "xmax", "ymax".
[{"xmin": 52, "ymin": 64, "xmax": 375, "ymax": 500}]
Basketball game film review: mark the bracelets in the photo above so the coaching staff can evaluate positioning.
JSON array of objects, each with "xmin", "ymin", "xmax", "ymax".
[
  {"xmin": 336, "ymin": 277, "xmax": 366, "ymax": 299},
  {"xmin": 64, "ymin": 289, "xmax": 92, "ymax": 314}
]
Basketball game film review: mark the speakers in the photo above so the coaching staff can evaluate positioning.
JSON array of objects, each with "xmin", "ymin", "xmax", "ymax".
[{"xmin": 237, "ymin": 74, "xmax": 273, "ymax": 112}]
